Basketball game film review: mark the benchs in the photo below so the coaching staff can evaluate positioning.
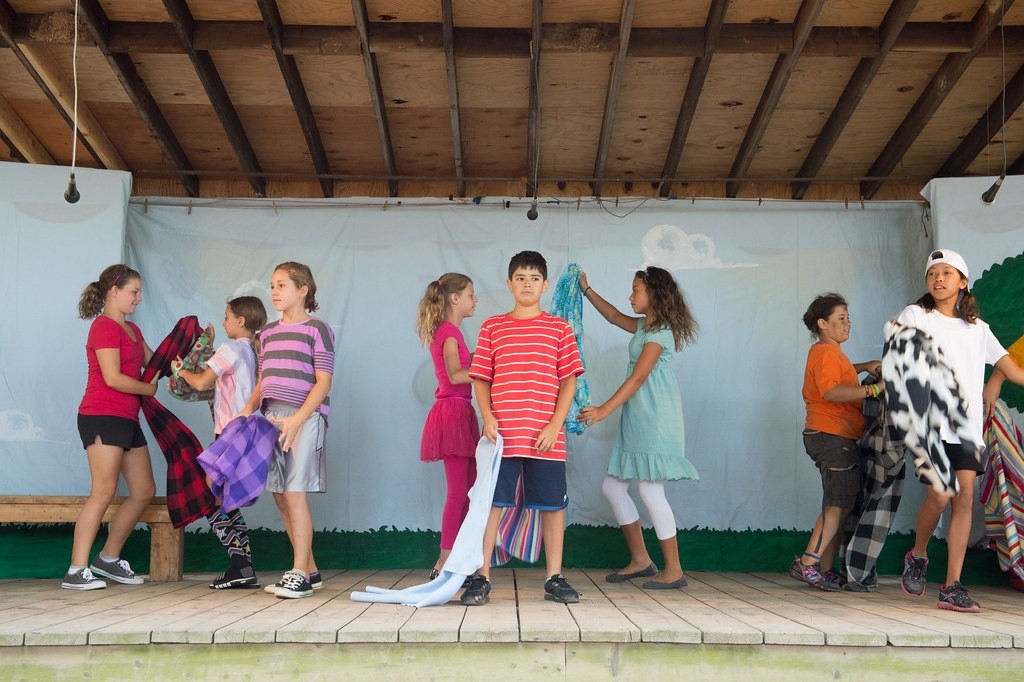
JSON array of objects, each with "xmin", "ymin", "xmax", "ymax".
[{"xmin": 0, "ymin": 495, "xmax": 184, "ymax": 582}]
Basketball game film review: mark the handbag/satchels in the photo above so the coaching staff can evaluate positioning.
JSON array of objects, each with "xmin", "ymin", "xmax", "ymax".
[{"xmin": 167, "ymin": 332, "xmax": 216, "ymax": 403}]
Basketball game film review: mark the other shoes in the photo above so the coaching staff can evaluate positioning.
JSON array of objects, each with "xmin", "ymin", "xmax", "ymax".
[
  {"xmin": 429, "ymin": 567, "xmax": 474, "ymax": 588},
  {"xmin": 606, "ymin": 561, "xmax": 659, "ymax": 582},
  {"xmin": 643, "ymin": 574, "xmax": 689, "ymax": 589}
]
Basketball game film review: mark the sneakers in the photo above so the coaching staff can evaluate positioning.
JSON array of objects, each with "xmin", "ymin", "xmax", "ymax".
[
  {"xmin": 60, "ymin": 568, "xmax": 107, "ymax": 590},
  {"xmin": 938, "ymin": 580, "xmax": 981, "ymax": 613},
  {"xmin": 90, "ymin": 553, "xmax": 145, "ymax": 584},
  {"xmin": 808, "ymin": 563, "xmax": 849, "ymax": 588},
  {"xmin": 900, "ymin": 548, "xmax": 929, "ymax": 599},
  {"xmin": 274, "ymin": 570, "xmax": 314, "ymax": 598},
  {"xmin": 460, "ymin": 575, "xmax": 492, "ymax": 606},
  {"xmin": 264, "ymin": 570, "xmax": 322, "ymax": 594},
  {"xmin": 544, "ymin": 574, "xmax": 580, "ymax": 603},
  {"xmin": 790, "ymin": 554, "xmax": 831, "ymax": 592},
  {"xmin": 208, "ymin": 559, "xmax": 260, "ymax": 589}
]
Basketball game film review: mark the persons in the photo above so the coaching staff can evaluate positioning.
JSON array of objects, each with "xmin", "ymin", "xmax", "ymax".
[
  {"xmin": 984, "ymin": 335, "xmax": 1024, "ymax": 417},
  {"xmin": 235, "ymin": 262, "xmax": 335, "ymax": 600},
  {"xmin": 577, "ymin": 266, "xmax": 699, "ymax": 590},
  {"xmin": 459, "ymin": 251, "xmax": 584, "ymax": 605},
  {"xmin": 790, "ymin": 292, "xmax": 885, "ymax": 591},
  {"xmin": 416, "ymin": 272, "xmax": 478, "ymax": 581},
  {"xmin": 891, "ymin": 249, "xmax": 1024, "ymax": 612},
  {"xmin": 61, "ymin": 264, "xmax": 161, "ymax": 590},
  {"xmin": 170, "ymin": 297, "xmax": 266, "ymax": 588}
]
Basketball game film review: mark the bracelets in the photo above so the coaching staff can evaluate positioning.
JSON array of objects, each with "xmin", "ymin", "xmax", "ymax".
[
  {"xmin": 584, "ymin": 287, "xmax": 591, "ymax": 296},
  {"xmin": 865, "ymin": 384, "xmax": 880, "ymax": 398}
]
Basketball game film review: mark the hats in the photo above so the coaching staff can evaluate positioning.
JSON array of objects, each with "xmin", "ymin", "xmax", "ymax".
[{"xmin": 924, "ymin": 248, "xmax": 969, "ymax": 280}]
